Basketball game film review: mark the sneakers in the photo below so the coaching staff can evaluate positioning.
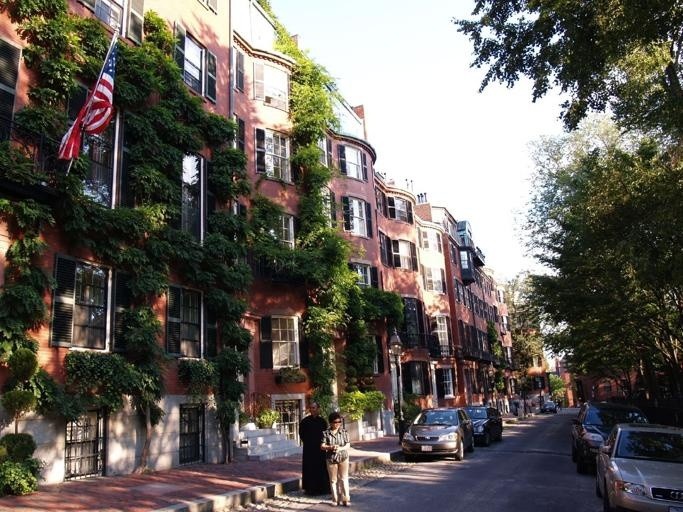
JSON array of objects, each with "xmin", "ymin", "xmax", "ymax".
[
  {"xmin": 330, "ymin": 502, "xmax": 339, "ymax": 506},
  {"xmin": 343, "ymin": 501, "xmax": 351, "ymax": 506}
]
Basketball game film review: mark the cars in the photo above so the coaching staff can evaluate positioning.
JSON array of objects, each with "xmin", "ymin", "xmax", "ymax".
[
  {"xmin": 571, "ymin": 400, "xmax": 682, "ymax": 511},
  {"xmin": 543, "ymin": 400, "xmax": 561, "ymax": 414},
  {"xmin": 402, "ymin": 407, "xmax": 504, "ymax": 459}
]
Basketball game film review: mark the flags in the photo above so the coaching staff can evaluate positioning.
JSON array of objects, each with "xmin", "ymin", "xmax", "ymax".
[{"xmin": 57, "ymin": 35, "xmax": 119, "ymax": 160}]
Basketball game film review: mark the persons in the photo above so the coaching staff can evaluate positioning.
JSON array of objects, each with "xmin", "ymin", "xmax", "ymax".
[
  {"xmin": 298, "ymin": 400, "xmax": 328, "ymax": 494},
  {"xmin": 322, "ymin": 413, "xmax": 352, "ymax": 508}
]
